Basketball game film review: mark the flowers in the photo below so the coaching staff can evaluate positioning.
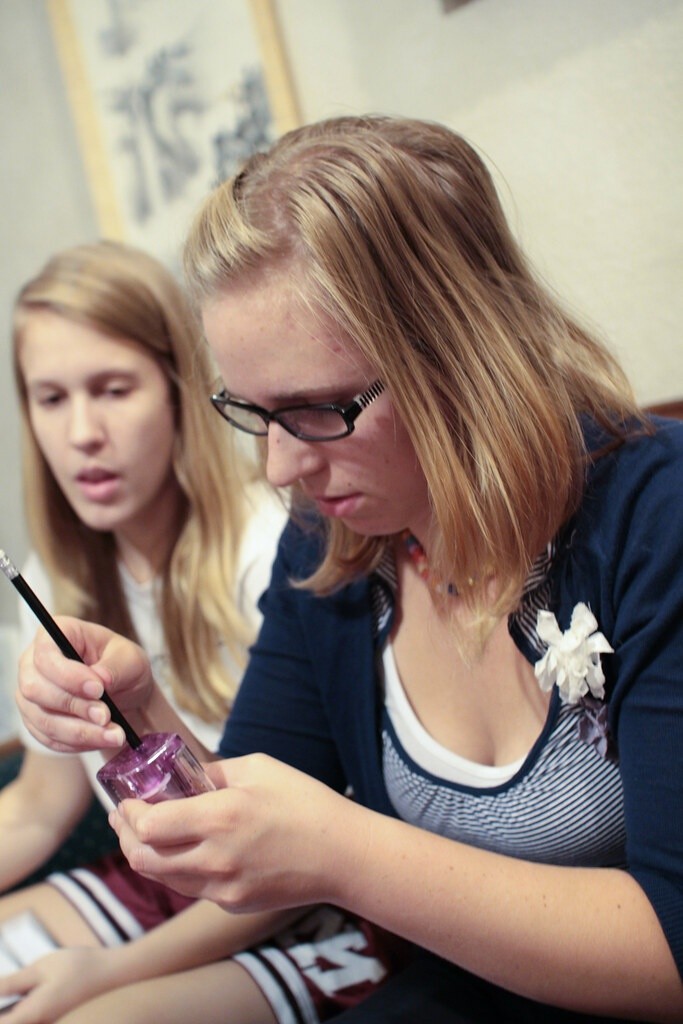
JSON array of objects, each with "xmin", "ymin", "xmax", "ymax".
[{"xmin": 532, "ymin": 600, "xmax": 616, "ymax": 706}]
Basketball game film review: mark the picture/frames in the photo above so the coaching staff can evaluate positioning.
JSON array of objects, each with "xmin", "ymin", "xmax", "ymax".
[{"xmin": 46, "ymin": 0, "xmax": 304, "ymax": 285}]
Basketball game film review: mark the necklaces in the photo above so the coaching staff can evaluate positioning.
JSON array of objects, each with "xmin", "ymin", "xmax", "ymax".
[{"xmin": 400, "ymin": 524, "xmax": 498, "ymax": 593}]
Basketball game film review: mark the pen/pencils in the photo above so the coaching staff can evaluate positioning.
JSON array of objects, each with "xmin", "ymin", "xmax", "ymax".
[{"xmin": 0, "ymin": 549, "xmax": 144, "ymax": 752}]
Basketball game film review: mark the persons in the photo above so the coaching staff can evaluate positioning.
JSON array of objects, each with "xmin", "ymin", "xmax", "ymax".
[
  {"xmin": 0, "ymin": 236, "xmax": 385, "ymax": 1024},
  {"xmin": 20, "ymin": 117, "xmax": 682, "ymax": 1022}
]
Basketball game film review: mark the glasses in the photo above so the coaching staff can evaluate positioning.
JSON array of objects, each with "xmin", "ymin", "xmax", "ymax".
[{"xmin": 209, "ymin": 375, "xmax": 386, "ymax": 441}]
